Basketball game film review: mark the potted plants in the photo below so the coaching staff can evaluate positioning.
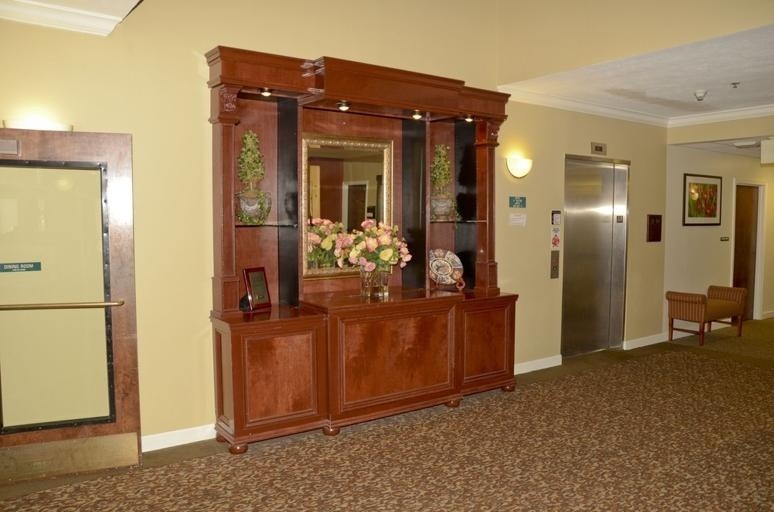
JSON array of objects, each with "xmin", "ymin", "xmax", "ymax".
[
  {"xmin": 234, "ymin": 129, "xmax": 273, "ymax": 223},
  {"xmin": 432, "ymin": 143, "xmax": 461, "ymax": 228}
]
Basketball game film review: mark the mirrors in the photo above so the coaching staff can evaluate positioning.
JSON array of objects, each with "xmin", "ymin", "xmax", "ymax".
[{"xmin": 301, "ymin": 137, "xmax": 394, "ymax": 281}]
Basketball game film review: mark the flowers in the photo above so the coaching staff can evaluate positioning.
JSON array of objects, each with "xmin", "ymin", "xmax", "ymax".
[
  {"xmin": 349, "ymin": 218, "xmax": 412, "ymax": 271},
  {"xmin": 306, "ymin": 217, "xmax": 349, "ymax": 269}
]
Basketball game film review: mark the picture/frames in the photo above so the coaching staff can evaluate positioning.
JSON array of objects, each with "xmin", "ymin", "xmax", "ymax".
[
  {"xmin": 681, "ymin": 173, "xmax": 723, "ymax": 226},
  {"xmin": 243, "ymin": 266, "xmax": 272, "ymax": 311}
]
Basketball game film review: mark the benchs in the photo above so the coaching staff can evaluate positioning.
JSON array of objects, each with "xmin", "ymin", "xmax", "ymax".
[{"xmin": 666, "ymin": 286, "xmax": 746, "ymax": 345}]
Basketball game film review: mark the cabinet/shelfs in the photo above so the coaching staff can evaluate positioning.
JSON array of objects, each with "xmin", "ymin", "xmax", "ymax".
[{"xmin": 205, "ymin": 45, "xmax": 520, "ymax": 455}]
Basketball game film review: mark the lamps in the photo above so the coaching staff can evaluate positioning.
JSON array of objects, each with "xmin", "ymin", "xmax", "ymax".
[{"xmin": 508, "ymin": 158, "xmax": 534, "ymax": 177}]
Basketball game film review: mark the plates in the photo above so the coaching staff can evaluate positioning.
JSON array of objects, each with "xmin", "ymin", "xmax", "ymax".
[{"xmin": 429, "ymin": 248, "xmax": 464, "ymax": 286}]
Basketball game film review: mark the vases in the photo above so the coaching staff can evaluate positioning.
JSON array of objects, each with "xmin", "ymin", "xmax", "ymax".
[{"xmin": 360, "ymin": 266, "xmax": 391, "ymax": 297}]
